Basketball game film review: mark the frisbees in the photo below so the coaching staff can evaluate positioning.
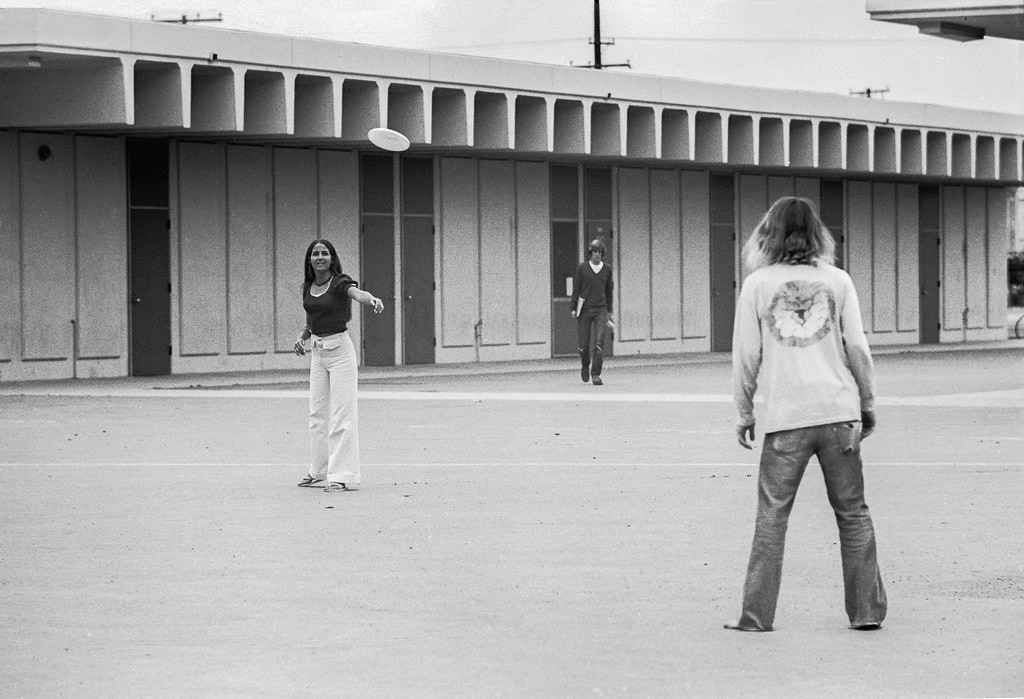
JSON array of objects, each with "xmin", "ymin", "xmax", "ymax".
[{"xmin": 368, "ymin": 128, "xmax": 411, "ymax": 151}]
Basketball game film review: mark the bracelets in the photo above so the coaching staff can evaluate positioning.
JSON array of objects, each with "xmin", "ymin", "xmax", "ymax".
[{"xmin": 302, "ymin": 327, "xmax": 312, "ymax": 338}]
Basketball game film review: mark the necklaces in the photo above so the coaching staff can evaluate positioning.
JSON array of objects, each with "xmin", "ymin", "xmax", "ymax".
[{"xmin": 313, "ymin": 274, "xmax": 332, "ymax": 285}]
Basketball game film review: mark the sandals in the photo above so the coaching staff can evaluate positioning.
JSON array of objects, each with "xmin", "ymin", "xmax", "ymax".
[
  {"xmin": 297, "ymin": 473, "xmax": 328, "ymax": 488},
  {"xmin": 324, "ymin": 483, "xmax": 350, "ymax": 493}
]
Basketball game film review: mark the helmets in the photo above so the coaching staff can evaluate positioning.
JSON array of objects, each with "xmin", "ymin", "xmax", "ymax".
[{"xmin": 589, "ymin": 240, "xmax": 607, "ymax": 256}]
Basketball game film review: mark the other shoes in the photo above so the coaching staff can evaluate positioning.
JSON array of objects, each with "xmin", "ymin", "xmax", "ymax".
[
  {"xmin": 592, "ymin": 375, "xmax": 603, "ymax": 386},
  {"xmin": 581, "ymin": 367, "xmax": 590, "ymax": 382},
  {"xmin": 724, "ymin": 617, "xmax": 745, "ymax": 630}
]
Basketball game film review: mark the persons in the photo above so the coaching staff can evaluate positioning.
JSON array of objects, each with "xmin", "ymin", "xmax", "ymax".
[
  {"xmin": 293, "ymin": 239, "xmax": 384, "ymax": 493},
  {"xmin": 570, "ymin": 239, "xmax": 614, "ymax": 385},
  {"xmin": 724, "ymin": 196, "xmax": 887, "ymax": 631}
]
